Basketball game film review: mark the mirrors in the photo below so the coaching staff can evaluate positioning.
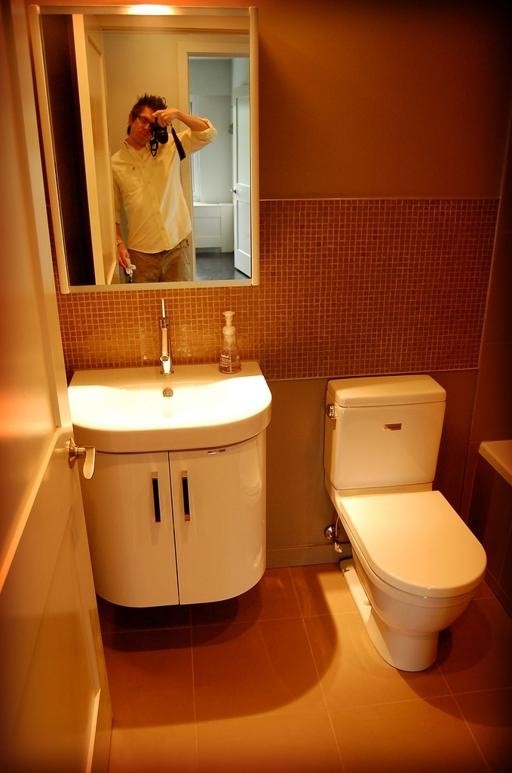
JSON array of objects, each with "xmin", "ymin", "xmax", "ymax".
[{"xmin": 28, "ymin": 6, "xmax": 261, "ymax": 295}]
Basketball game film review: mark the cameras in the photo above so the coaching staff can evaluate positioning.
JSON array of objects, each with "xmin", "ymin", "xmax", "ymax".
[{"xmin": 149, "ymin": 117, "xmax": 169, "ymax": 146}]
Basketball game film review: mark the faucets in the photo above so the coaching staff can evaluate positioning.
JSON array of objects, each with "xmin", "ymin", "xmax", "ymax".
[{"xmin": 155, "ymin": 296, "xmax": 174, "ymax": 378}]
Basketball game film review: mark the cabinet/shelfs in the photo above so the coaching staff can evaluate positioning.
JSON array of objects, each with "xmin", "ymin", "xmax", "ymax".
[{"xmin": 74, "ymin": 428, "xmax": 267, "ymax": 609}]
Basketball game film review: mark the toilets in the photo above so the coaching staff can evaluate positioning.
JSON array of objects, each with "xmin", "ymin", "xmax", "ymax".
[{"xmin": 320, "ymin": 373, "xmax": 488, "ymax": 675}]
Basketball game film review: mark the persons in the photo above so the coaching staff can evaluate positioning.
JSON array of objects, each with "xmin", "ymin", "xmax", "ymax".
[{"xmin": 110, "ymin": 93, "xmax": 218, "ymax": 284}]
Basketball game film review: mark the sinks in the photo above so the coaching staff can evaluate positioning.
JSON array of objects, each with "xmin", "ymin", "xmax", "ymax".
[{"xmin": 96, "ymin": 378, "xmax": 249, "ymax": 427}]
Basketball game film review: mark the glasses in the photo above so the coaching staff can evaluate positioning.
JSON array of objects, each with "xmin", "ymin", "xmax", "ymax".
[{"xmin": 137, "ymin": 113, "xmax": 154, "ymax": 126}]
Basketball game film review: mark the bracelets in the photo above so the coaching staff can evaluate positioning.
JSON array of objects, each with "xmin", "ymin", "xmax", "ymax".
[{"xmin": 116, "ymin": 239, "xmax": 124, "ymax": 248}]
